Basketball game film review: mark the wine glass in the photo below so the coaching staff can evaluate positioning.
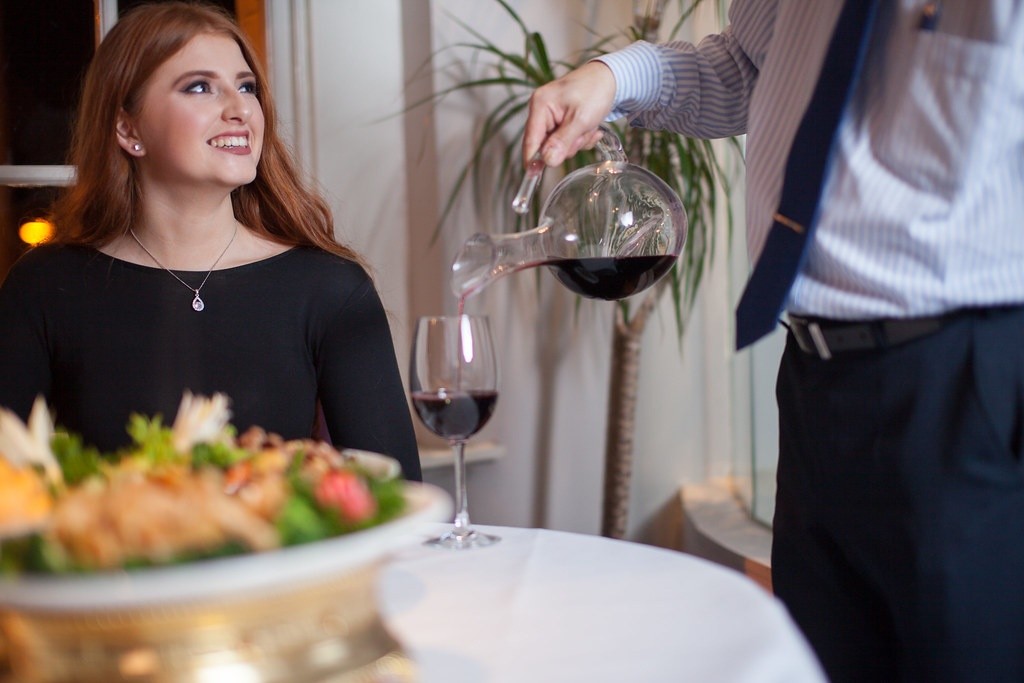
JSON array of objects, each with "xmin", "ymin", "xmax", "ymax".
[{"xmin": 408, "ymin": 315, "xmax": 502, "ymax": 550}]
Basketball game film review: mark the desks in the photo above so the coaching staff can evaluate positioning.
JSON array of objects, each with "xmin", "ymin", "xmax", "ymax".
[{"xmin": 376, "ymin": 524, "xmax": 830, "ymax": 683}]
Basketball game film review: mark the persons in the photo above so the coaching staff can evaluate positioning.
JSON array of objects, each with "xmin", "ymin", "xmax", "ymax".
[
  {"xmin": 1, "ymin": 3, "xmax": 422, "ymax": 482},
  {"xmin": 520, "ymin": 1, "xmax": 1024, "ymax": 683}
]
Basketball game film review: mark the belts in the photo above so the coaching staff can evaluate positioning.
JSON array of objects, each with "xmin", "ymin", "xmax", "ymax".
[{"xmin": 785, "ymin": 313, "xmax": 940, "ymax": 360}]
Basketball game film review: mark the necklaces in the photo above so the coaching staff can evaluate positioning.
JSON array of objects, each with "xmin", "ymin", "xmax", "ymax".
[{"xmin": 130, "ymin": 225, "xmax": 238, "ymax": 311}]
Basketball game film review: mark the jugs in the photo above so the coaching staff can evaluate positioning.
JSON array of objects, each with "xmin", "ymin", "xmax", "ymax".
[{"xmin": 450, "ymin": 118, "xmax": 688, "ymax": 302}]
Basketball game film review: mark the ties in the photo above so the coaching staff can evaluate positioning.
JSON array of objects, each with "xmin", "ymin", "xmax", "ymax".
[{"xmin": 735, "ymin": 0, "xmax": 878, "ymax": 351}]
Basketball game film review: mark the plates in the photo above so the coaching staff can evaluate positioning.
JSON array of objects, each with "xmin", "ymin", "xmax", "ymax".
[{"xmin": 0, "ymin": 483, "xmax": 454, "ymax": 613}]
